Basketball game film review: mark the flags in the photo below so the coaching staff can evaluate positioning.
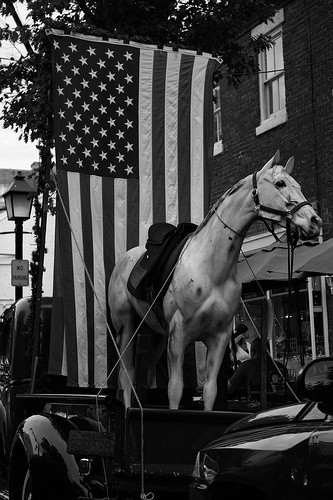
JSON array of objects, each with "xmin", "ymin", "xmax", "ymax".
[{"xmin": 51, "ymin": 29, "xmax": 217, "ymax": 390}]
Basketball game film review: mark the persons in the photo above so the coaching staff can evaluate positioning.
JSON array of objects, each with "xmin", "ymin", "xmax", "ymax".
[{"xmin": 221, "ymin": 319, "xmax": 305, "ymax": 411}]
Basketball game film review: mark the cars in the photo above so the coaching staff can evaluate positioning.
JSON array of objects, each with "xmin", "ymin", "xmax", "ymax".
[{"xmin": 185, "ymin": 356, "xmax": 333, "ymax": 500}]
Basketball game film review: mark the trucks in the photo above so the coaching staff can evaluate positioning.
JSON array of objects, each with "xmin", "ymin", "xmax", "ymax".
[{"xmin": 1, "ymin": 296, "xmax": 257, "ymax": 500}]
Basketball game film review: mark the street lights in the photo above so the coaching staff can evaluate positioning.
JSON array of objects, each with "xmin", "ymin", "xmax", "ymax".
[{"xmin": 0, "ymin": 170, "xmax": 38, "ymax": 302}]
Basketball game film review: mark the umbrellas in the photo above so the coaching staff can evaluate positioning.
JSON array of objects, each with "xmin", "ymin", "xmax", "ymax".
[{"xmin": 235, "ymin": 226, "xmax": 333, "ymax": 284}]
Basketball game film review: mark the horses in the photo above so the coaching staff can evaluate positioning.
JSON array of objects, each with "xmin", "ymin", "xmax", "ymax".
[{"xmin": 108, "ymin": 149, "xmax": 324, "ymax": 411}]
